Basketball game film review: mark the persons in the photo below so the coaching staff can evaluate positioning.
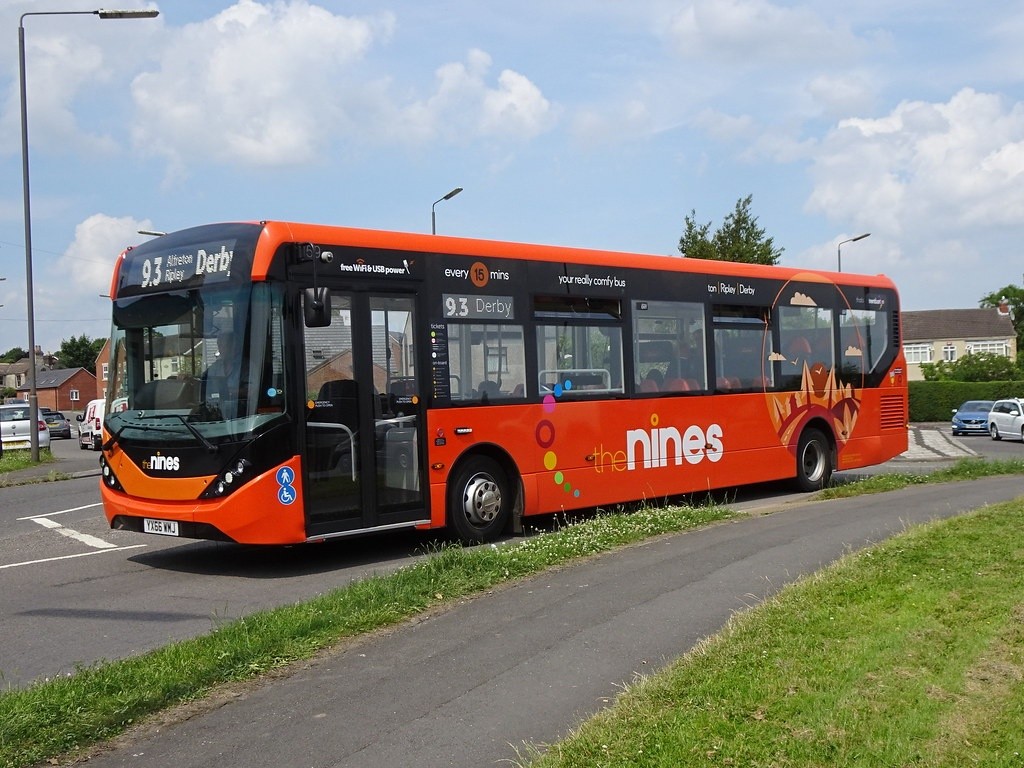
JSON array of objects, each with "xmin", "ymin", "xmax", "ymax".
[
  {"xmin": 178, "ymin": 330, "xmax": 264, "ymax": 417},
  {"xmin": 666, "ymin": 329, "xmax": 771, "ymax": 381}
]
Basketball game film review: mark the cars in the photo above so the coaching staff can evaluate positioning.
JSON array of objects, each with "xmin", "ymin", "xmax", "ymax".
[
  {"xmin": 0, "ymin": 404, "xmax": 49, "ymax": 452},
  {"xmin": 43, "ymin": 412, "xmax": 71, "ymax": 439},
  {"xmin": 76, "ymin": 399, "xmax": 106, "ymax": 451},
  {"xmin": 22, "ymin": 407, "xmax": 51, "ymax": 421},
  {"xmin": 951, "ymin": 400, "xmax": 997, "ymax": 437},
  {"xmin": 987, "ymin": 398, "xmax": 1024, "ymax": 443}
]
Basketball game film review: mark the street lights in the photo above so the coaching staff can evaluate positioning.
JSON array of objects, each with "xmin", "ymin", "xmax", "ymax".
[
  {"xmin": 19, "ymin": 7, "xmax": 159, "ymax": 457},
  {"xmin": 431, "ymin": 187, "xmax": 464, "ymax": 235},
  {"xmin": 837, "ymin": 234, "xmax": 871, "ymax": 273}
]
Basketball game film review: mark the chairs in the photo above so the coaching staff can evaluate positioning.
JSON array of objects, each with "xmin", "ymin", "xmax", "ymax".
[
  {"xmin": 55, "ymin": 416, "xmax": 60, "ymax": 419},
  {"xmin": 635, "ymin": 330, "xmax": 868, "ymax": 394},
  {"xmin": 22, "ymin": 411, "xmax": 30, "ymax": 419},
  {"xmin": 303, "ymin": 378, "xmax": 362, "ymax": 471},
  {"xmin": 475, "ymin": 379, "xmax": 553, "ymax": 397}
]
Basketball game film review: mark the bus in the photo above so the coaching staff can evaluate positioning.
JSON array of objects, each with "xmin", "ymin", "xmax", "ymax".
[{"xmin": 99, "ymin": 221, "xmax": 910, "ymax": 550}]
